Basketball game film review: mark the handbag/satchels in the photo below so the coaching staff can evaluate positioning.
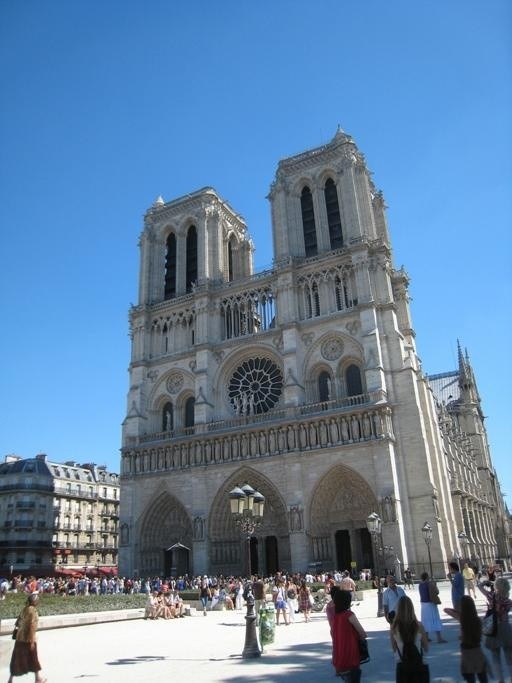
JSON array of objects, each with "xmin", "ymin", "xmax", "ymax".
[
  {"xmin": 482, "ymin": 609, "xmax": 498, "ymax": 637},
  {"xmin": 359, "ymin": 640, "xmax": 370, "ymax": 663}
]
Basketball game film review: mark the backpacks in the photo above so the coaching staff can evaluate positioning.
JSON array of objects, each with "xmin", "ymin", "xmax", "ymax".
[{"xmin": 396, "ymin": 643, "xmax": 430, "ymax": 683}]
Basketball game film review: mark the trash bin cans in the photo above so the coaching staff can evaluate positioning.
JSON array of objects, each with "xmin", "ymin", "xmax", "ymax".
[
  {"xmin": 259, "ymin": 608, "xmax": 276, "ymax": 643},
  {"xmin": 494, "ymin": 560, "xmax": 507, "ymax": 574},
  {"xmin": 360, "ymin": 569, "xmax": 371, "ymax": 581}
]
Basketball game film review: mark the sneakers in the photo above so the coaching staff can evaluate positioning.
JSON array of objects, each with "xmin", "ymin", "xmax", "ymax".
[
  {"xmin": 204, "ymin": 612, "xmax": 207, "ymax": 616},
  {"xmin": 150, "ymin": 614, "xmax": 185, "ymax": 619},
  {"xmin": 276, "ymin": 619, "xmax": 312, "ymax": 626},
  {"xmin": 427, "ymin": 638, "xmax": 432, "ymax": 642},
  {"xmin": 438, "ymin": 640, "xmax": 448, "ymax": 644},
  {"xmin": 404, "ymin": 587, "xmax": 415, "ymax": 590}
]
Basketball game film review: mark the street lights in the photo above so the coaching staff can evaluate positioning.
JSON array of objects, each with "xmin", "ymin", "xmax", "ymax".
[
  {"xmin": 228, "ymin": 478, "xmax": 266, "ymax": 658},
  {"xmin": 422, "ymin": 520, "xmax": 436, "ymax": 582},
  {"xmin": 458, "ymin": 532, "xmax": 469, "ymax": 560},
  {"xmin": 367, "ymin": 509, "xmax": 389, "ymax": 617},
  {"xmin": 378, "ymin": 543, "xmax": 394, "ymax": 576}
]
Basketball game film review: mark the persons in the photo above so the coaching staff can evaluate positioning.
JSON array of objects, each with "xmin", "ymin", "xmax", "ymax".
[
  {"xmin": 484, "ymin": 564, "xmax": 496, "ymax": 592},
  {"xmin": 0, "ymin": 567, "xmax": 252, "ymax": 620},
  {"xmin": 442, "ymin": 595, "xmax": 489, "ymax": 682},
  {"xmin": 251, "ymin": 568, "xmax": 413, "ymax": 626},
  {"xmin": 417, "ymin": 570, "xmax": 449, "ymax": 644},
  {"xmin": 331, "ymin": 588, "xmax": 368, "ymax": 682},
  {"xmin": 8, "ymin": 593, "xmax": 48, "ymax": 683},
  {"xmin": 389, "ymin": 595, "xmax": 431, "ymax": 682},
  {"xmin": 326, "ymin": 585, "xmax": 345, "ymax": 677},
  {"xmin": 462, "ymin": 561, "xmax": 478, "ymax": 599},
  {"xmin": 445, "ymin": 560, "xmax": 468, "ymax": 641},
  {"xmin": 477, "ymin": 577, "xmax": 511, "ymax": 682}
]
